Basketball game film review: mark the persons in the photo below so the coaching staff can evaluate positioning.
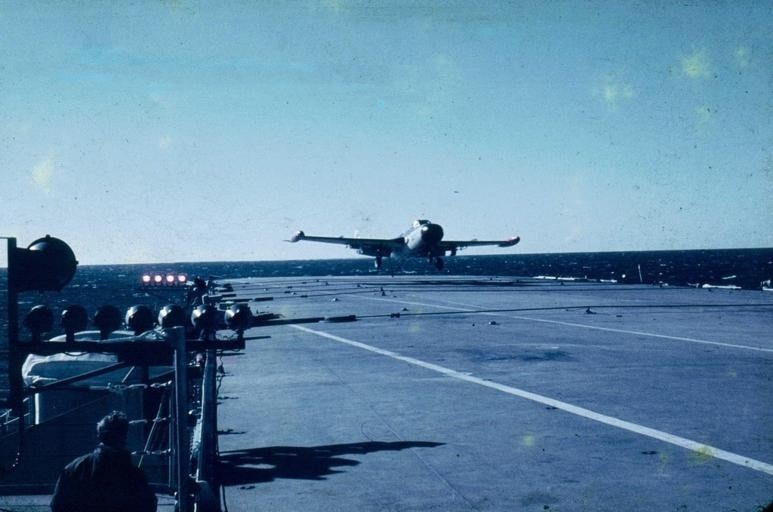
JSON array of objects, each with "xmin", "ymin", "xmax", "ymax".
[{"xmin": 49, "ymin": 410, "xmax": 158, "ymax": 512}]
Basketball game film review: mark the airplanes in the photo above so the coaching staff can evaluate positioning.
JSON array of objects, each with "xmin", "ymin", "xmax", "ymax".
[{"xmin": 284, "ymin": 216, "xmax": 520, "ymax": 272}]
